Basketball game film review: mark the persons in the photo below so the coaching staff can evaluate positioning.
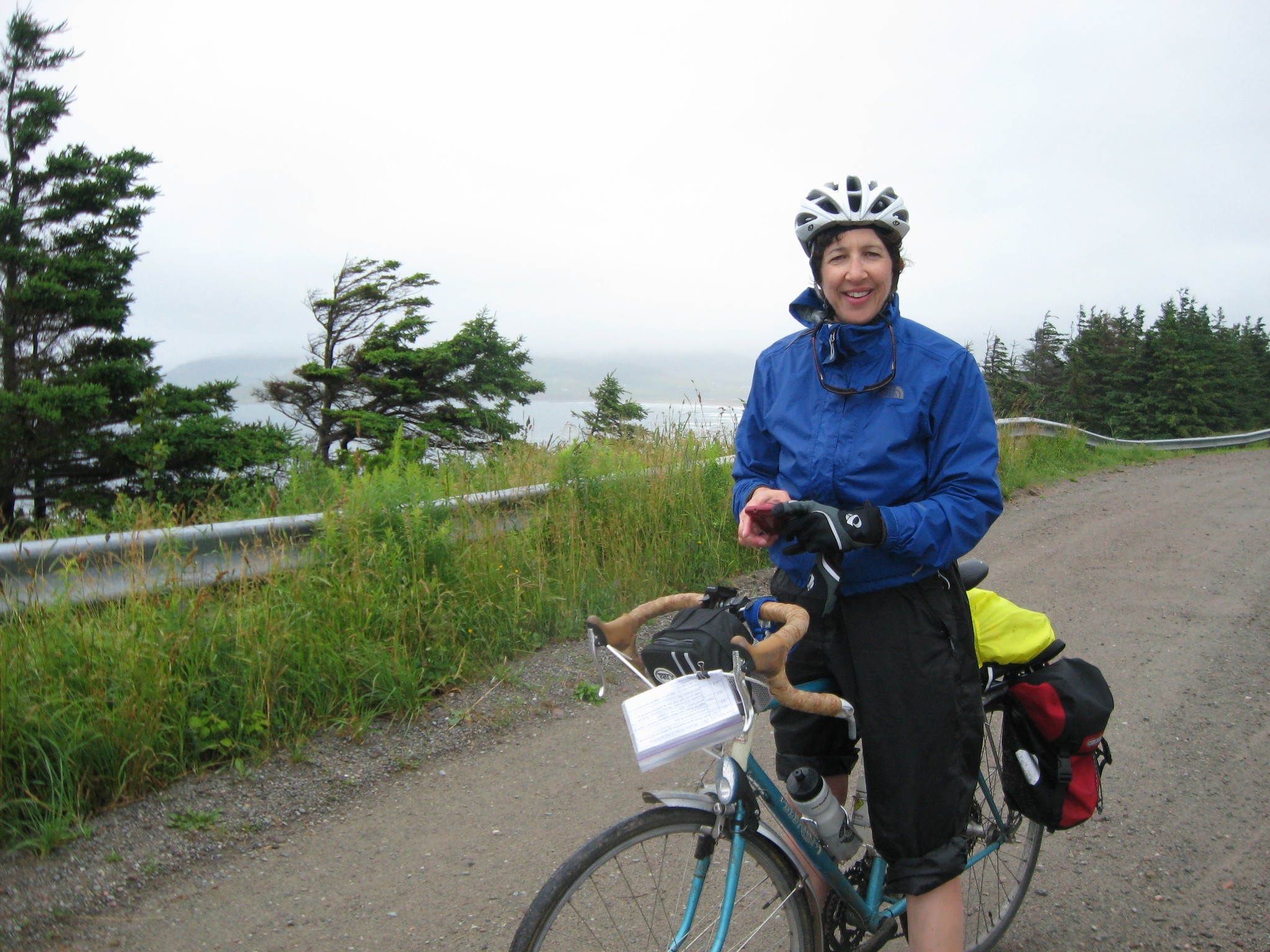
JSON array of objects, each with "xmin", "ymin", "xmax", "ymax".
[{"xmin": 731, "ymin": 170, "xmax": 1004, "ymax": 952}]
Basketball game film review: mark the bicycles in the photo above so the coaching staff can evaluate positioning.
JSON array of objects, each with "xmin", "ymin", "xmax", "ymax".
[{"xmin": 504, "ymin": 558, "xmax": 1046, "ymax": 952}]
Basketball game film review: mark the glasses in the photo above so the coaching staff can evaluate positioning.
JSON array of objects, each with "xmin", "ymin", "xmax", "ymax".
[{"xmin": 812, "ymin": 313, "xmax": 896, "ymax": 394}]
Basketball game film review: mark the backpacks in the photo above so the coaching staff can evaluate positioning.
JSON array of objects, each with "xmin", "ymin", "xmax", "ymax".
[
  {"xmin": 641, "ymin": 600, "xmax": 756, "ymax": 715},
  {"xmin": 1002, "ymin": 658, "xmax": 1114, "ymax": 833}
]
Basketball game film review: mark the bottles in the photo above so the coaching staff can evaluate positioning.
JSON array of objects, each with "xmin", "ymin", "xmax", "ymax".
[
  {"xmin": 786, "ymin": 765, "xmax": 860, "ymax": 861},
  {"xmin": 851, "ymin": 755, "xmax": 873, "ymax": 847}
]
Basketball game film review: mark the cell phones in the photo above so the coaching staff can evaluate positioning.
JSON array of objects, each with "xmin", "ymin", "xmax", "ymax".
[{"xmin": 744, "ymin": 504, "xmax": 782, "ymax": 536}]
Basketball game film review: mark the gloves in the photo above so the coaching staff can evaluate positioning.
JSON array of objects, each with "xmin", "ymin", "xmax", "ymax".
[
  {"xmin": 791, "ymin": 554, "xmax": 842, "ymax": 628},
  {"xmin": 771, "ymin": 501, "xmax": 886, "ymax": 554}
]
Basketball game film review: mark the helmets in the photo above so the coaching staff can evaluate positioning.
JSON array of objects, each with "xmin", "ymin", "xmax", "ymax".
[{"xmin": 794, "ymin": 176, "xmax": 910, "ymax": 258}]
